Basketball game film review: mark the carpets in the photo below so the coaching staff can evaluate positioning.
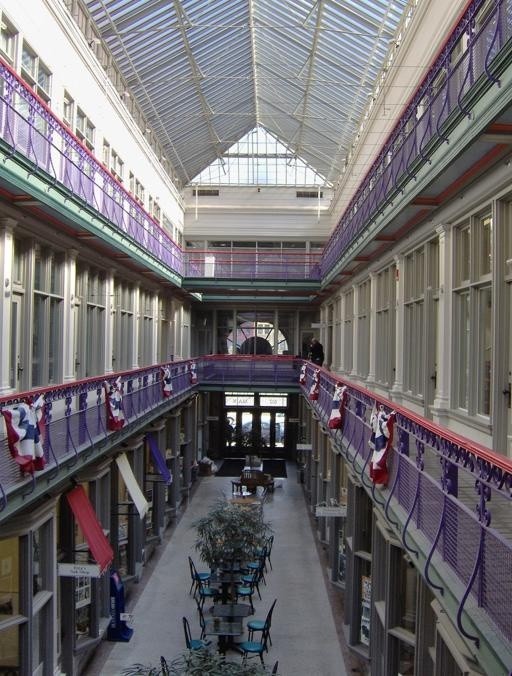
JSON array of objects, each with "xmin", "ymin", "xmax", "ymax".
[{"xmin": 214, "ymin": 458, "xmax": 288, "ymax": 478}]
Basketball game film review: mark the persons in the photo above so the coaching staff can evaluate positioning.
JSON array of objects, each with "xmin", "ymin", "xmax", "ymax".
[{"xmin": 309, "ymin": 337, "xmax": 324, "ymax": 368}]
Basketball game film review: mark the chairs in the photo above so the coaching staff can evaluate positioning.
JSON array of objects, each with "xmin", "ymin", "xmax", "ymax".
[
  {"xmin": 182, "ymin": 617, "xmax": 211, "ymax": 656},
  {"xmin": 240, "ymin": 598, "xmax": 279, "ymax": 668},
  {"xmin": 159, "ymin": 656, "xmax": 171, "ymax": 676},
  {"xmin": 188, "ymin": 535, "xmax": 276, "ymax": 613},
  {"xmin": 230, "ymin": 468, "xmax": 275, "ymax": 494}
]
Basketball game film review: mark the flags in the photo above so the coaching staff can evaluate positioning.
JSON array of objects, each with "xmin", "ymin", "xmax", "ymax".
[
  {"xmin": 368, "ymin": 401, "xmax": 397, "ymax": 486},
  {"xmin": 308, "ymin": 369, "xmax": 320, "ymax": 401},
  {"xmin": 103, "ymin": 376, "xmax": 127, "ymax": 433},
  {"xmin": 298, "ymin": 363, "xmax": 306, "ymax": 385},
  {"xmin": 159, "ymin": 364, "xmax": 173, "ymax": 398},
  {"xmin": 325, "ymin": 384, "xmax": 348, "ymax": 430},
  {"xmin": 2, "ymin": 393, "xmax": 47, "ymax": 474},
  {"xmin": 189, "ymin": 359, "xmax": 198, "ymax": 384}
]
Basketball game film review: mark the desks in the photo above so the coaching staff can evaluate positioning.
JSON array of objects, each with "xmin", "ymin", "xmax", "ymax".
[{"xmin": 202, "ymin": 601, "xmax": 255, "ymax": 657}]
